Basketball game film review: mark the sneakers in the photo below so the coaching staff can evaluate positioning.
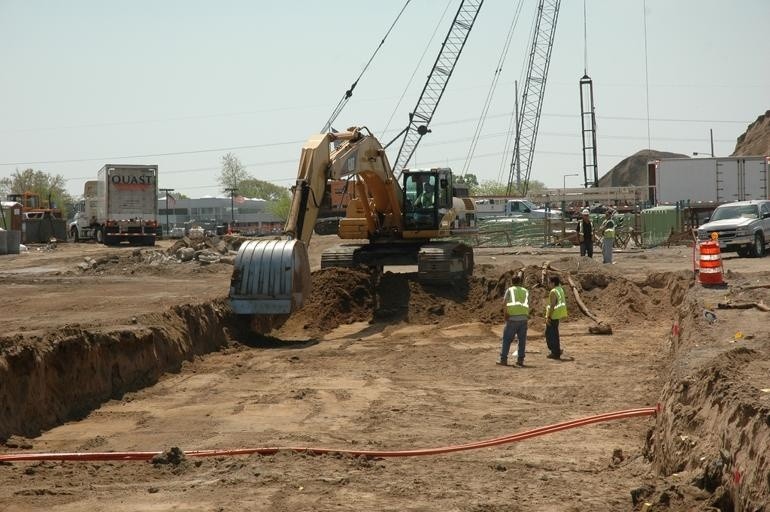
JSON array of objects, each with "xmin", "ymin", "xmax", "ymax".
[
  {"xmin": 516, "ymin": 361, "xmax": 522, "ymax": 366},
  {"xmin": 496, "ymin": 360, "xmax": 508, "ymax": 366},
  {"xmin": 546, "ymin": 354, "xmax": 560, "ymax": 359}
]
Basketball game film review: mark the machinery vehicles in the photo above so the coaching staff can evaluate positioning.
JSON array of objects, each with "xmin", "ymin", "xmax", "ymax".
[
  {"xmin": 5, "ymin": 191, "xmax": 68, "ymax": 244},
  {"xmin": 224, "ymin": 126, "xmax": 481, "ymax": 319}
]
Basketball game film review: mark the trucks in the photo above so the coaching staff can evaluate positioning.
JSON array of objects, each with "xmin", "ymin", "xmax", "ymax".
[{"xmin": 69, "ymin": 163, "xmax": 159, "ymax": 247}]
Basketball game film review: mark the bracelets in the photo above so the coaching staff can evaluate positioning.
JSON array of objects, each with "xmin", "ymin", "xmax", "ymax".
[{"xmin": 548, "ymin": 316, "xmax": 551, "ymax": 319}]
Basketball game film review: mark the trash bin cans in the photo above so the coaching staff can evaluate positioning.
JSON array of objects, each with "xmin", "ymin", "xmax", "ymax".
[{"xmin": 22, "ymin": 212, "xmax": 44, "ymax": 244}]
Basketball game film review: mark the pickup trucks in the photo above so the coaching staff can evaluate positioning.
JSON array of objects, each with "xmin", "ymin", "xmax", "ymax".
[{"xmin": 476, "ymin": 199, "xmax": 563, "ymax": 221}]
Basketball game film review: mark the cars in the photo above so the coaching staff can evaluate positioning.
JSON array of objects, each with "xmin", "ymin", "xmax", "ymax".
[{"xmin": 170, "ymin": 222, "xmax": 204, "ymax": 238}]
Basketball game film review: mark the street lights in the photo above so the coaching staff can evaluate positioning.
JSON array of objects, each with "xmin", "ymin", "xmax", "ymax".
[
  {"xmin": 225, "ymin": 188, "xmax": 239, "ymax": 220},
  {"xmin": 159, "ymin": 188, "xmax": 174, "ymax": 236},
  {"xmin": 693, "ymin": 152, "xmax": 714, "ymax": 158},
  {"xmin": 562, "ymin": 172, "xmax": 579, "ymax": 201}
]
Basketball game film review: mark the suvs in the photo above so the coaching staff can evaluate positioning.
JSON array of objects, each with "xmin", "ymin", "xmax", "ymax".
[{"xmin": 696, "ymin": 198, "xmax": 770, "ymax": 257}]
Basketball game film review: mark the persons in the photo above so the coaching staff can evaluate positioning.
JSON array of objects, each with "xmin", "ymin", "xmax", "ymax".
[
  {"xmin": 543, "ymin": 275, "xmax": 567, "ymax": 358},
  {"xmin": 599, "ymin": 210, "xmax": 615, "ymax": 263},
  {"xmin": 496, "ymin": 275, "xmax": 530, "ymax": 367},
  {"xmin": 412, "ymin": 184, "xmax": 437, "ymax": 228},
  {"xmin": 576, "ymin": 209, "xmax": 593, "ymax": 258}
]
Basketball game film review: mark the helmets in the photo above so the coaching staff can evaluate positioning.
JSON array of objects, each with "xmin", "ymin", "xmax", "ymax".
[{"xmin": 582, "ymin": 209, "xmax": 589, "ymax": 216}]
